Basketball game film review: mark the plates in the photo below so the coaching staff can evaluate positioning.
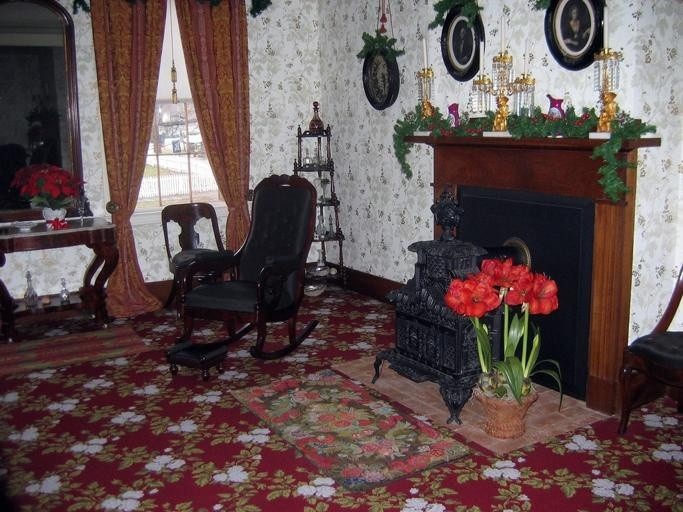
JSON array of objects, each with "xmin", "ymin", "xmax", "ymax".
[{"xmin": 11, "ymin": 221, "xmax": 38, "ymax": 231}]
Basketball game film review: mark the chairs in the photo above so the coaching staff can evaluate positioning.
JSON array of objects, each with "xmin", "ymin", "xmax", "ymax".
[
  {"xmin": 615, "ymin": 263, "xmax": 683, "ymax": 436},
  {"xmin": 172, "ymin": 172, "xmax": 321, "ymax": 360},
  {"xmin": 160, "ymin": 202, "xmax": 242, "ymax": 323}
]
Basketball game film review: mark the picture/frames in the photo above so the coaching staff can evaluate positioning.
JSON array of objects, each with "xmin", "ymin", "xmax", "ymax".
[
  {"xmin": 439, "ymin": 4, "xmax": 486, "ymax": 84},
  {"xmin": 361, "ymin": 50, "xmax": 400, "ymax": 111},
  {"xmin": 542, "ymin": 0, "xmax": 605, "ymax": 72}
]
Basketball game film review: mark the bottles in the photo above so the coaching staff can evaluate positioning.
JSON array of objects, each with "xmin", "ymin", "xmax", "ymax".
[
  {"xmin": 448, "ymin": 103, "xmax": 461, "ymax": 127},
  {"xmin": 22, "ymin": 270, "xmax": 38, "ymax": 306},
  {"xmin": 307, "ymin": 101, "xmax": 324, "ymax": 134},
  {"xmin": 316, "ymin": 215, "xmax": 325, "ymax": 235},
  {"xmin": 327, "ymin": 214, "xmax": 335, "ymax": 237},
  {"xmin": 304, "ymin": 145, "xmax": 326, "ymax": 168},
  {"xmin": 544, "ymin": 95, "xmax": 563, "ymax": 135},
  {"xmin": 57, "ymin": 277, "xmax": 69, "ymax": 303}
]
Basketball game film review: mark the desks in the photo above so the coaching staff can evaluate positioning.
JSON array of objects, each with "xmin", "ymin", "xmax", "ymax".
[{"xmin": 0, "ymin": 217, "xmax": 122, "ymax": 344}]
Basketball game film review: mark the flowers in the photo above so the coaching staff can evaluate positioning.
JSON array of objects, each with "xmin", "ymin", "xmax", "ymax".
[
  {"xmin": 18, "ymin": 165, "xmax": 87, "ymax": 207},
  {"xmin": 7, "ymin": 166, "xmax": 47, "ymax": 189},
  {"xmin": 443, "ymin": 258, "xmax": 565, "ymax": 411}
]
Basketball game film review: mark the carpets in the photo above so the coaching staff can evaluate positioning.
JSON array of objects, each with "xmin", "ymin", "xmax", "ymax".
[
  {"xmin": 330, "ymin": 348, "xmax": 610, "ymax": 458},
  {"xmin": 0, "ymin": 324, "xmax": 149, "ymax": 377},
  {"xmin": 224, "ymin": 369, "xmax": 473, "ymax": 494}
]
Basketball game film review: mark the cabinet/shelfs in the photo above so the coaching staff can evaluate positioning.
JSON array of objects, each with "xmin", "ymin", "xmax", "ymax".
[{"xmin": 290, "ymin": 122, "xmax": 346, "ymax": 288}]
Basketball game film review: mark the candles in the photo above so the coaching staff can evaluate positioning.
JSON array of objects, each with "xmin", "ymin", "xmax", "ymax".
[
  {"xmin": 499, "ymin": 14, "xmax": 506, "ymax": 57},
  {"xmin": 524, "ymin": 37, "xmax": 529, "ymax": 82},
  {"xmin": 478, "ymin": 40, "xmax": 485, "ymax": 77},
  {"xmin": 421, "ymin": 37, "xmax": 427, "ymax": 73},
  {"xmin": 602, "ymin": 6, "xmax": 609, "ymax": 51}
]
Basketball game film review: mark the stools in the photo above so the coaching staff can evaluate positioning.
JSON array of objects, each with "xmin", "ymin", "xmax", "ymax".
[{"xmin": 166, "ymin": 341, "xmax": 228, "ymax": 381}]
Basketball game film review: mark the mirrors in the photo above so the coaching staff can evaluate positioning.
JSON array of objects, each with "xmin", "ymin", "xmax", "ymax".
[{"xmin": 1, "ymin": 1, "xmax": 86, "ymax": 219}]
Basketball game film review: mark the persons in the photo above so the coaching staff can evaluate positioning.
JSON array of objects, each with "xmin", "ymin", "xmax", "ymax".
[
  {"xmin": 455, "ymin": 27, "xmax": 469, "ymax": 64},
  {"xmin": 563, "ymin": 5, "xmax": 591, "ymax": 50}
]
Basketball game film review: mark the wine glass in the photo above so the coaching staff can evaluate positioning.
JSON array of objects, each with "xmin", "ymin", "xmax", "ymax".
[
  {"xmin": 319, "ymin": 181, "xmax": 329, "ymax": 204},
  {"xmin": 315, "ymin": 249, "xmax": 324, "ymax": 267},
  {"xmin": 74, "ymin": 201, "xmax": 88, "ymax": 223}
]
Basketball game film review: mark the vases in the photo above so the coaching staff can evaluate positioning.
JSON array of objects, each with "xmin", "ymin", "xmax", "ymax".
[
  {"xmin": 40, "ymin": 206, "xmax": 68, "ymax": 222},
  {"xmin": 466, "ymin": 382, "xmax": 538, "ymax": 440}
]
